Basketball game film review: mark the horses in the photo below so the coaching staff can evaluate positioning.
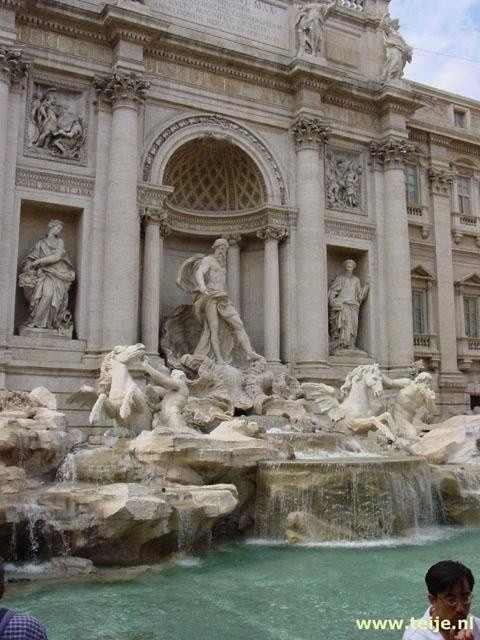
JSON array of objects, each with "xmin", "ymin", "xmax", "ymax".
[
  {"xmin": 88, "ymin": 342, "xmax": 153, "ymax": 436},
  {"xmin": 295, "ymin": 363, "xmax": 399, "ymax": 442}
]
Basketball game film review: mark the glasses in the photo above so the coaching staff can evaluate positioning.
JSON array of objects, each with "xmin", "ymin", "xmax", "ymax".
[{"xmin": 436, "ymin": 592, "xmax": 471, "ymax": 604}]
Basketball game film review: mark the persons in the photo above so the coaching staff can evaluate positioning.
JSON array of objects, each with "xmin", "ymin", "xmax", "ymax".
[
  {"xmin": 0, "ymin": 556, "xmax": 51, "ymax": 640},
  {"xmin": 325, "ymin": 157, "xmax": 361, "ymax": 209},
  {"xmin": 402, "ymin": 560, "xmax": 480, "ymax": 640},
  {"xmin": 381, "ymin": 370, "xmax": 438, "ymax": 437},
  {"xmin": 32, "ymin": 86, "xmax": 85, "ymax": 158},
  {"xmin": 142, "ymin": 352, "xmax": 192, "ymax": 428},
  {"xmin": 328, "ymin": 259, "xmax": 369, "ymax": 345},
  {"xmin": 196, "ymin": 239, "xmax": 263, "ymax": 364},
  {"xmin": 301, "ymin": 0, "xmax": 336, "ymax": 57},
  {"xmin": 380, "ymin": 12, "xmax": 407, "ymax": 81},
  {"xmin": 25, "ymin": 219, "xmax": 76, "ymax": 326}
]
